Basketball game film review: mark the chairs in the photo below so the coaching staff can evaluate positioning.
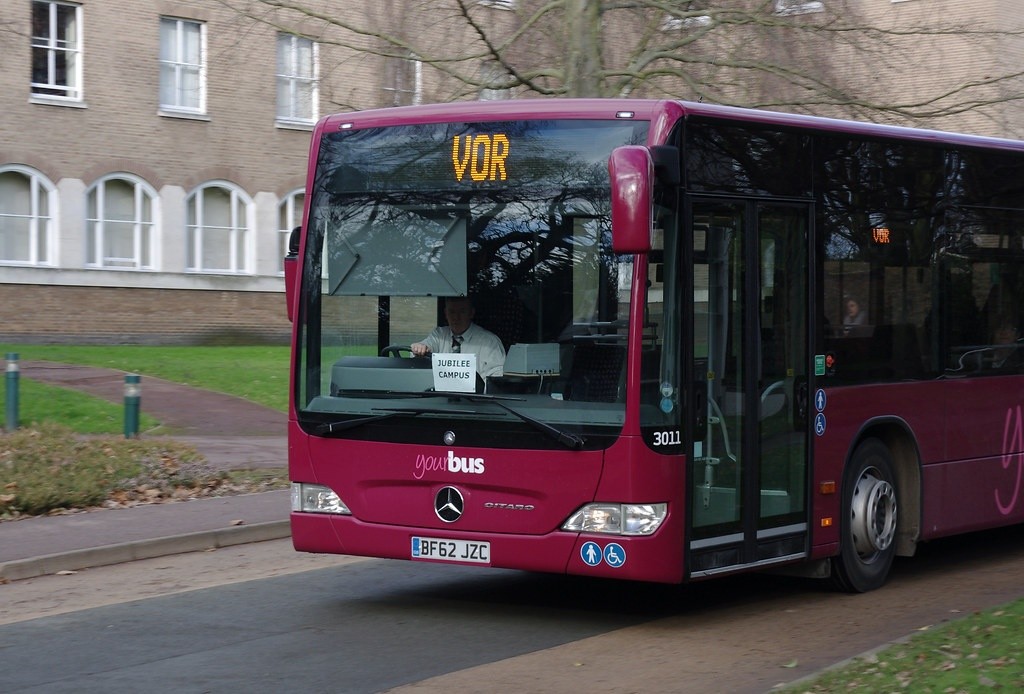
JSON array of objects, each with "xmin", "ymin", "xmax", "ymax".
[{"xmin": 739, "ymin": 309, "xmax": 1023, "ymax": 384}]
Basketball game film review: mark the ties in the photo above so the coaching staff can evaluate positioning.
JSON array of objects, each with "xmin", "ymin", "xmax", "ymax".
[{"xmin": 451, "ymin": 336, "xmax": 463, "ymax": 354}]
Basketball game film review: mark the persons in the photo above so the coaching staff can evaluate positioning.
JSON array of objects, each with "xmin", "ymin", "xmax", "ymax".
[
  {"xmin": 923, "ymin": 266, "xmax": 978, "ymax": 351},
  {"xmin": 410, "ymin": 286, "xmax": 508, "ymax": 405},
  {"xmin": 974, "ymin": 307, "xmax": 1024, "ymax": 377},
  {"xmin": 842, "ymin": 297, "xmax": 872, "ymax": 340}
]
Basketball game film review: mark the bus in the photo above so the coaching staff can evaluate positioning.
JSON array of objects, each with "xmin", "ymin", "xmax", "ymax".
[{"xmin": 282, "ymin": 98, "xmax": 1024, "ymax": 595}]
